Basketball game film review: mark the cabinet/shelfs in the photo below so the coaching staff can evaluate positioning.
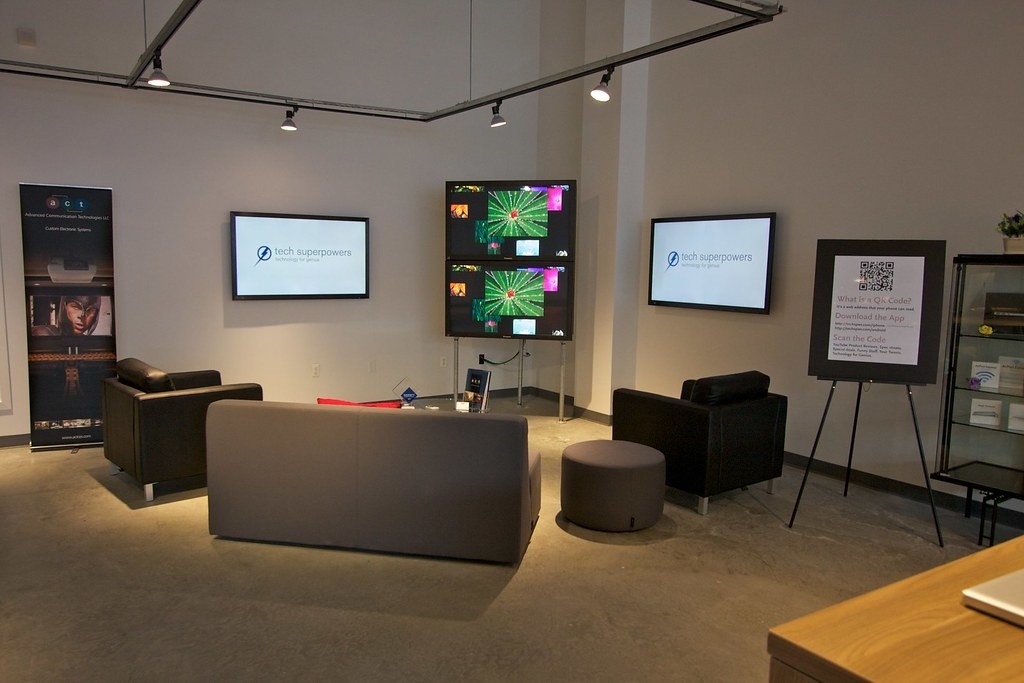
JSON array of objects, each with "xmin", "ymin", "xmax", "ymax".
[{"xmin": 929, "ymin": 252, "xmax": 1024, "ymax": 548}]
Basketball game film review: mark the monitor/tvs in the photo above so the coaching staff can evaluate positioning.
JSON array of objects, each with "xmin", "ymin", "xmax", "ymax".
[
  {"xmin": 647, "ymin": 212, "xmax": 776, "ymax": 315},
  {"xmin": 230, "ymin": 210, "xmax": 369, "ymax": 302},
  {"xmin": 445, "ymin": 179, "xmax": 578, "ymax": 341}
]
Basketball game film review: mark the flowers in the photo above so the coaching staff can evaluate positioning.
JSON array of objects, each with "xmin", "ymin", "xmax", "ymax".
[{"xmin": 995, "ymin": 208, "xmax": 1024, "ymax": 238}]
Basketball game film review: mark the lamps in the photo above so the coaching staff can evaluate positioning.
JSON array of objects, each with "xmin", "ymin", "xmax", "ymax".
[
  {"xmin": 148, "ymin": 50, "xmax": 171, "ymax": 88},
  {"xmin": 590, "ymin": 66, "xmax": 615, "ymax": 102},
  {"xmin": 490, "ymin": 99, "xmax": 507, "ymax": 128},
  {"xmin": 280, "ymin": 106, "xmax": 300, "ymax": 131}
]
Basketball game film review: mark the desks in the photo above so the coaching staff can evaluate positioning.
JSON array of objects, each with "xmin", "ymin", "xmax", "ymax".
[{"xmin": 763, "ymin": 533, "xmax": 1024, "ymax": 683}]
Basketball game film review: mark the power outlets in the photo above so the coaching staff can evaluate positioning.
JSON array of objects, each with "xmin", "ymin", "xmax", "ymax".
[
  {"xmin": 440, "ymin": 357, "xmax": 447, "ymax": 368},
  {"xmin": 479, "ymin": 354, "xmax": 484, "ymax": 364}
]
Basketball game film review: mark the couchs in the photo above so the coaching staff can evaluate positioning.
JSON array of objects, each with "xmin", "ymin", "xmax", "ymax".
[
  {"xmin": 612, "ymin": 370, "xmax": 788, "ymax": 515},
  {"xmin": 104, "ymin": 357, "xmax": 263, "ymax": 503},
  {"xmin": 206, "ymin": 399, "xmax": 543, "ymax": 566}
]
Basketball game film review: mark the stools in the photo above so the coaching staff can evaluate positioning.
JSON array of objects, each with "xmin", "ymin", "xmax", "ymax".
[{"xmin": 561, "ymin": 440, "xmax": 667, "ymax": 533}]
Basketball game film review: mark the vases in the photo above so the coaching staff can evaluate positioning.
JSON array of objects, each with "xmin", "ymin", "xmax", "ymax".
[{"xmin": 1002, "ymin": 238, "xmax": 1024, "ymax": 254}]
